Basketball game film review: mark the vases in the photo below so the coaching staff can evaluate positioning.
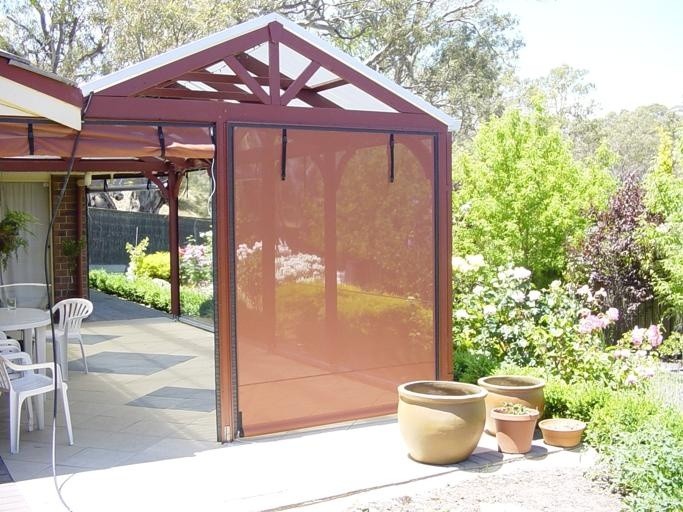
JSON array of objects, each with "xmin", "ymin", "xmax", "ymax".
[
  {"xmin": 477, "ymin": 375, "xmax": 545, "ymax": 437},
  {"xmin": 398, "ymin": 381, "xmax": 488, "ymax": 465}
]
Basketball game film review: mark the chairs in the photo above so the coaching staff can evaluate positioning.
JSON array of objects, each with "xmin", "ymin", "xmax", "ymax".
[{"xmin": 0, "ymin": 298, "xmax": 93, "ymax": 455}]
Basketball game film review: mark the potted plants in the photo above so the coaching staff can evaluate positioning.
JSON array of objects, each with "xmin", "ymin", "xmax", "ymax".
[
  {"xmin": 491, "ymin": 402, "xmax": 540, "ymax": 454},
  {"xmin": 539, "ymin": 418, "xmax": 585, "ymax": 447},
  {"xmin": 0, "ymin": 211, "xmax": 45, "ymax": 273}
]
